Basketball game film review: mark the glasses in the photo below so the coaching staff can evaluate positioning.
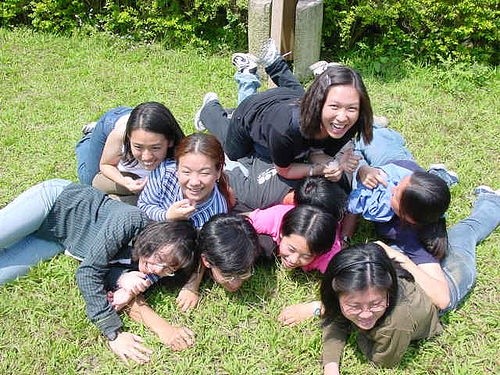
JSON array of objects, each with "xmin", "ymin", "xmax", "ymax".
[
  {"xmin": 341, "ymin": 290, "xmax": 389, "ymax": 315},
  {"xmin": 153, "ymin": 251, "xmax": 175, "ymax": 277},
  {"xmin": 212, "ymin": 260, "xmax": 255, "ymax": 282}
]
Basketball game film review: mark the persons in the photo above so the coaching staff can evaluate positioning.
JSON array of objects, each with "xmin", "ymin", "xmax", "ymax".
[{"xmin": 0, "ymin": 37, "xmax": 500, "ymax": 375}]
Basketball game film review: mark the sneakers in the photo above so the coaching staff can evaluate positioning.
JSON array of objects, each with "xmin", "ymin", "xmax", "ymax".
[
  {"xmin": 194, "ymin": 92, "xmax": 219, "ymax": 133},
  {"xmin": 231, "ymin": 52, "xmax": 260, "ymax": 73},
  {"xmin": 256, "ymin": 38, "xmax": 277, "ymax": 67}
]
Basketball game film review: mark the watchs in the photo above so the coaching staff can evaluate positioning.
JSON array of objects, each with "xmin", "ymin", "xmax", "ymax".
[
  {"xmin": 105, "ymin": 328, "xmax": 123, "ymax": 341},
  {"xmin": 341, "ymin": 235, "xmax": 351, "ymax": 245}
]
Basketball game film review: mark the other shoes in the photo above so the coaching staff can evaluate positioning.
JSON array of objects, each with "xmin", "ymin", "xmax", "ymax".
[
  {"xmin": 372, "ymin": 114, "xmax": 391, "ymax": 129},
  {"xmin": 309, "ymin": 60, "xmax": 328, "ymax": 75},
  {"xmin": 81, "ymin": 120, "xmax": 97, "ymax": 133},
  {"xmin": 430, "ymin": 163, "xmax": 460, "ymax": 184},
  {"xmin": 475, "ymin": 185, "xmax": 500, "ymax": 200}
]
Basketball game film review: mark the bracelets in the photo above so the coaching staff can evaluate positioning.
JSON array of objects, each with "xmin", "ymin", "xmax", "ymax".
[
  {"xmin": 311, "ymin": 301, "xmax": 322, "ymax": 317},
  {"xmin": 309, "ymin": 163, "xmax": 316, "ymax": 176}
]
